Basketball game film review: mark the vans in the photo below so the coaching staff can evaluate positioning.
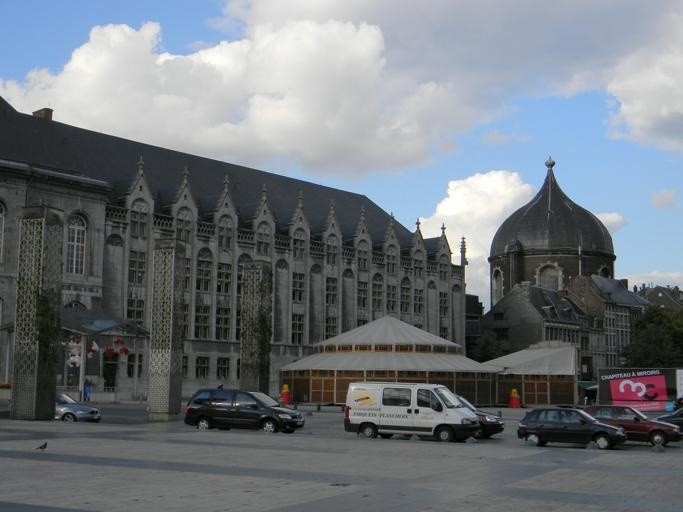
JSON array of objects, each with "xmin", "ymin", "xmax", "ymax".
[{"xmin": 343, "ymin": 381, "xmax": 481, "ymax": 442}]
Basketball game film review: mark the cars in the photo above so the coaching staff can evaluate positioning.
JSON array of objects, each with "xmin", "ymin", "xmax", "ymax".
[
  {"xmin": 456, "ymin": 394, "xmax": 504, "ymax": 439},
  {"xmin": 55, "ymin": 392, "xmax": 101, "ymax": 422},
  {"xmin": 184, "ymin": 388, "xmax": 305, "ymax": 433},
  {"xmin": 517, "ymin": 396, "xmax": 683, "ymax": 449}
]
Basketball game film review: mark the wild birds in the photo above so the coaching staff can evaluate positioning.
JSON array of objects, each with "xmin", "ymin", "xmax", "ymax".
[{"xmin": 36, "ymin": 442, "xmax": 47, "ymax": 450}]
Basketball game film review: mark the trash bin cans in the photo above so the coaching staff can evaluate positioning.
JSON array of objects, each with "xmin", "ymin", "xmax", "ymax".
[
  {"xmin": 509, "ymin": 389, "xmax": 520, "ymax": 408},
  {"xmin": 280, "ymin": 384, "xmax": 290, "ymax": 404}
]
